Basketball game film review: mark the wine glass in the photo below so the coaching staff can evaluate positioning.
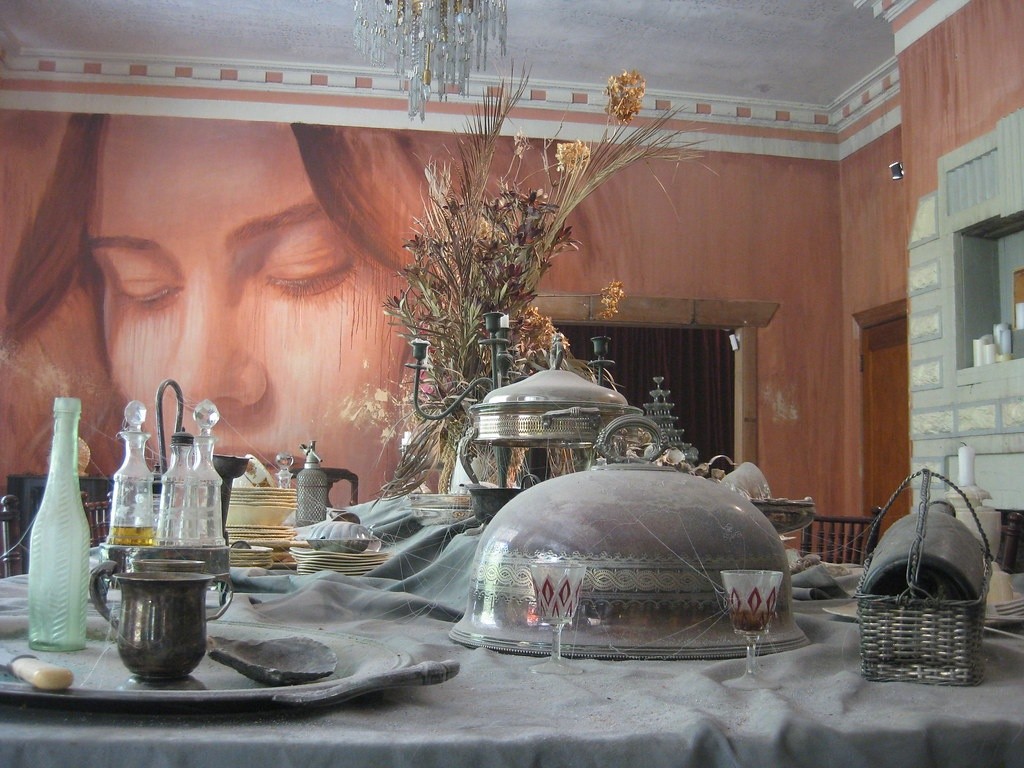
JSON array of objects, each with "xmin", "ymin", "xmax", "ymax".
[
  {"xmin": 529, "ymin": 564, "xmax": 586, "ymax": 675},
  {"xmin": 721, "ymin": 568, "xmax": 783, "ymax": 691}
]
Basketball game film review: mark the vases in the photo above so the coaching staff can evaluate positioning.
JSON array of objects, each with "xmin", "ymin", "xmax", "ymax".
[{"xmin": 445, "ymin": 434, "xmax": 516, "ymax": 499}]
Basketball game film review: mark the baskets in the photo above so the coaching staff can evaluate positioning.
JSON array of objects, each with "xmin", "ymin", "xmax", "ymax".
[{"xmin": 853, "ymin": 469, "xmax": 991, "ymax": 686}]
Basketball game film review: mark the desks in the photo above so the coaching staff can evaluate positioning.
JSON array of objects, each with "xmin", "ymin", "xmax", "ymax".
[{"xmin": 0, "ymin": 551, "xmax": 1024, "ymax": 768}]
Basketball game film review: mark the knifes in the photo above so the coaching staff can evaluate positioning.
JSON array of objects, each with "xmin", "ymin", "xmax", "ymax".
[{"xmin": 0, "ymin": 648, "xmax": 73, "ymax": 690}]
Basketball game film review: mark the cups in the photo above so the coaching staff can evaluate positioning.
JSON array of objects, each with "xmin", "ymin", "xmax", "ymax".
[
  {"xmin": 90, "ymin": 561, "xmax": 233, "ymax": 681},
  {"xmin": 973, "ymin": 339, "xmax": 986, "ymax": 366},
  {"xmin": 984, "ymin": 344, "xmax": 997, "ymax": 364},
  {"xmin": 131, "ymin": 558, "xmax": 205, "ymax": 576}
]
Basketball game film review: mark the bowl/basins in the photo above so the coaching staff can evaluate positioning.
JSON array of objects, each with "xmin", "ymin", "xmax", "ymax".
[{"xmin": 227, "ymin": 487, "xmax": 298, "ymax": 525}]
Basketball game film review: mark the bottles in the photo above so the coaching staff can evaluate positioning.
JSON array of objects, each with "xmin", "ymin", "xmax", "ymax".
[
  {"xmin": 862, "ymin": 498, "xmax": 985, "ymax": 600},
  {"xmin": 156, "ymin": 428, "xmax": 201, "ymax": 548},
  {"xmin": 193, "ymin": 399, "xmax": 226, "ymax": 546},
  {"xmin": 30, "ymin": 398, "xmax": 90, "ymax": 653},
  {"xmin": 108, "ymin": 400, "xmax": 156, "ymax": 546}
]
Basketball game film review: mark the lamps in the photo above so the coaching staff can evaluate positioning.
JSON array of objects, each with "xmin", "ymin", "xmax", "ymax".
[
  {"xmin": 725, "ymin": 327, "xmax": 741, "ymax": 352},
  {"xmin": 888, "ymin": 161, "xmax": 904, "ymax": 180}
]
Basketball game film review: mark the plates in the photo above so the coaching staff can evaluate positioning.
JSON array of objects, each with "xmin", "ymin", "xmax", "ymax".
[
  {"xmin": 993, "ymin": 592, "xmax": 1024, "ymax": 617},
  {"xmin": 225, "ymin": 524, "xmax": 389, "ymax": 575},
  {"xmin": 0, "ymin": 616, "xmax": 460, "ymax": 704}
]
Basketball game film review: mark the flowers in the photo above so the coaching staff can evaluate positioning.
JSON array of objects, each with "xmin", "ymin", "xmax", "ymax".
[{"xmin": 338, "ymin": 69, "xmax": 725, "ymax": 496}]
